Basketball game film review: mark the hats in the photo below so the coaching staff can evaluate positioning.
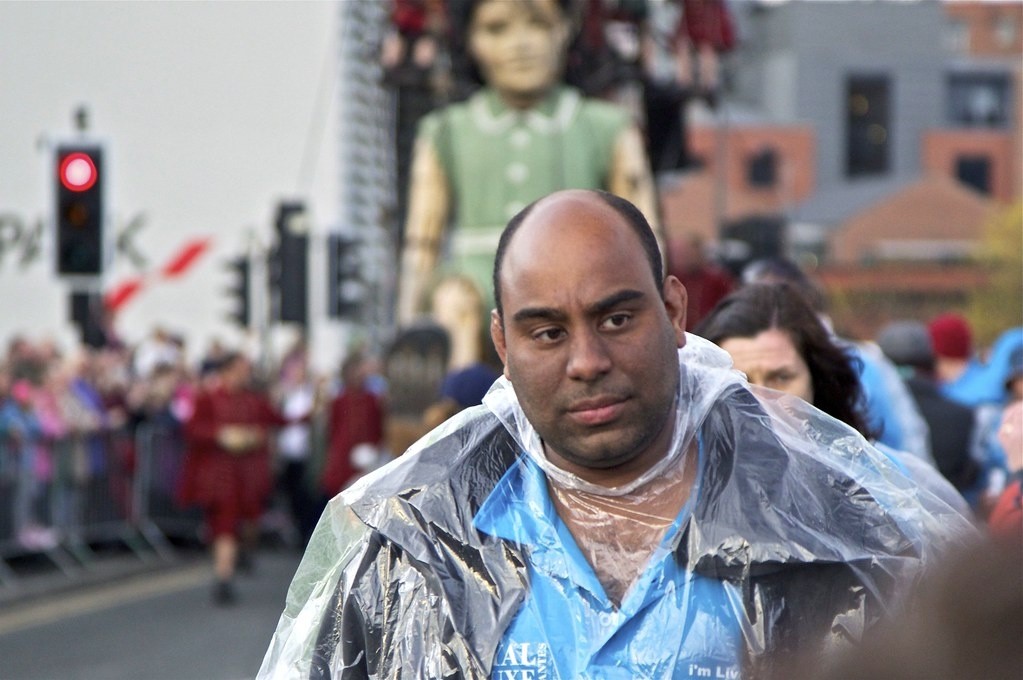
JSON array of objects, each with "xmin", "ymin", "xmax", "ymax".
[
  {"xmin": 876, "ymin": 321, "xmax": 930, "ymax": 361},
  {"xmin": 928, "ymin": 315, "xmax": 973, "ymax": 354}
]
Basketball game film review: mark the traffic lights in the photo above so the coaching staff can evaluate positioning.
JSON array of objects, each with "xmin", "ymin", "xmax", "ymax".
[
  {"xmin": 54, "ymin": 146, "xmax": 104, "ymax": 276},
  {"xmin": 223, "ymin": 248, "xmax": 252, "ymax": 328},
  {"xmin": 270, "ymin": 202, "xmax": 314, "ymax": 326}
]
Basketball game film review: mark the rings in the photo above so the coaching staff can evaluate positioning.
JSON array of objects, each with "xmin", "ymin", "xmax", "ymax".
[{"xmin": 1003, "ymin": 424, "xmax": 1013, "ymax": 434}]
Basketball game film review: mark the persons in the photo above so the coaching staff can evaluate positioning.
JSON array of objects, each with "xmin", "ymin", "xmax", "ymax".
[
  {"xmin": 739, "ymin": 255, "xmax": 931, "ymax": 462},
  {"xmin": 0, "ymin": 331, "xmax": 384, "ymax": 604},
  {"xmin": 257, "ymin": 189, "xmax": 981, "ymax": 680},
  {"xmin": 674, "ymin": 0, "xmax": 736, "ymax": 88},
  {"xmin": 381, "ymin": 1, "xmax": 440, "ymax": 69},
  {"xmin": 690, "ymin": 280, "xmax": 882, "ymax": 446},
  {"xmin": 396, "ymin": 0, "xmax": 665, "ymax": 306},
  {"xmin": 876, "ymin": 312, "xmax": 1023, "ymax": 532}
]
